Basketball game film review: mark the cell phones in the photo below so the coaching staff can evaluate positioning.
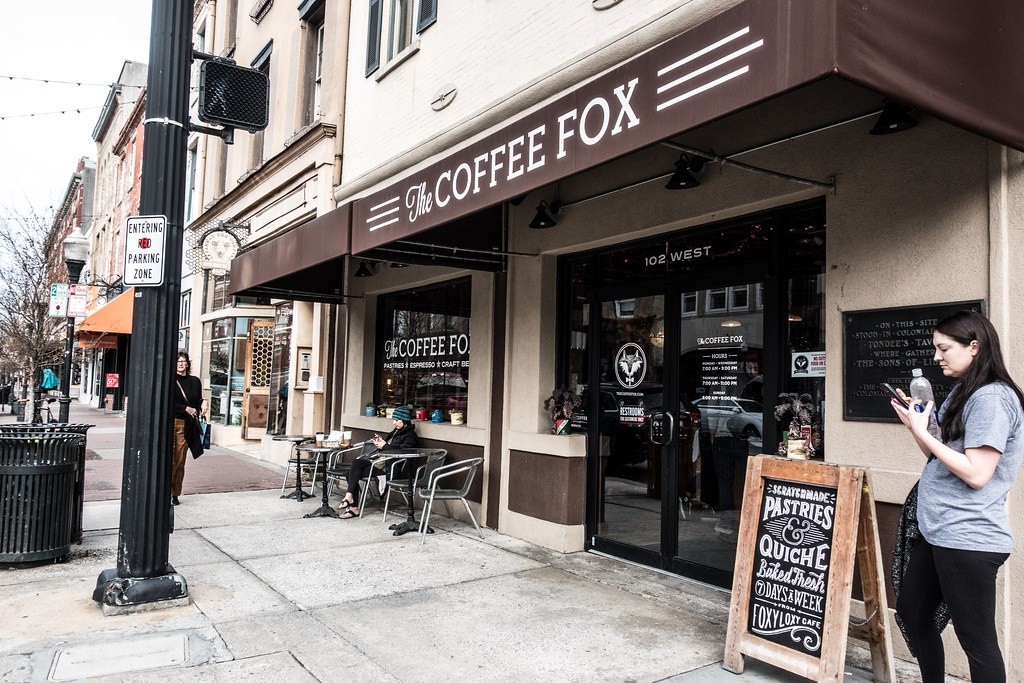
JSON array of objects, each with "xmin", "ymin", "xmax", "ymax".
[{"xmin": 879, "ymin": 383, "xmax": 909, "ymax": 411}]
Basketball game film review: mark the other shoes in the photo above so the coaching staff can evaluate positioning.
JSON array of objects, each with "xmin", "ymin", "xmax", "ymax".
[{"xmin": 172, "ymin": 496, "xmax": 180, "ymax": 505}]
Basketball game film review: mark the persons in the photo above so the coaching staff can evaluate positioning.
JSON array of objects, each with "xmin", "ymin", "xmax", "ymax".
[
  {"xmin": 895, "ymin": 309, "xmax": 1024, "ymax": 683},
  {"xmin": 336, "ymin": 405, "xmax": 416, "ymax": 519},
  {"xmin": 172, "ymin": 351, "xmax": 209, "ymax": 506}
]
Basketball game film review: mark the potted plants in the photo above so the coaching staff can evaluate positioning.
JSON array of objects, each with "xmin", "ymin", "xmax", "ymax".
[
  {"xmin": 416, "ymin": 407, "xmax": 427, "ymax": 421},
  {"xmin": 365, "ymin": 402, "xmax": 376, "ymax": 416},
  {"xmin": 386, "ymin": 405, "xmax": 397, "ymax": 419},
  {"xmin": 448, "ymin": 408, "xmax": 464, "ymax": 425},
  {"xmin": 377, "ymin": 403, "xmax": 389, "ymax": 417},
  {"xmin": 432, "ymin": 409, "xmax": 444, "ymax": 423},
  {"xmin": 543, "ymin": 389, "xmax": 582, "ymax": 435}
]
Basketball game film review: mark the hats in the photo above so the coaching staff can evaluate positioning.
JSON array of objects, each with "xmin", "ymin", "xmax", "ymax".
[{"xmin": 392, "ymin": 406, "xmax": 412, "ymax": 420}]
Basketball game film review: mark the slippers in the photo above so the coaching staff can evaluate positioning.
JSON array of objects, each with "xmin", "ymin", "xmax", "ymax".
[
  {"xmin": 338, "ymin": 510, "xmax": 359, "ymax": 519},
  {"xmin": 337, "ymin": 499, "xmax": 354, "ymax": 509}
]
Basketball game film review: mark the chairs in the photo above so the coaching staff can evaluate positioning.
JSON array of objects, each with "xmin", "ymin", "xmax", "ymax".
[{"xmin": 271, "ymin": 434, "xmax": 484, "ymax": 545}]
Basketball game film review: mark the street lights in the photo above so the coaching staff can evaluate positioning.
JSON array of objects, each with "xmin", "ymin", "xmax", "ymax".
[{"xmin": 54, "ymin": 223, "xmax": 93, "ymax": 426}]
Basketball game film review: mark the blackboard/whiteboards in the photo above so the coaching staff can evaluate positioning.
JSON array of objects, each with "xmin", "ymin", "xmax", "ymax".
[{"xmin": 739, "ymin": 453, "xmax": 862, "ymax": 683}]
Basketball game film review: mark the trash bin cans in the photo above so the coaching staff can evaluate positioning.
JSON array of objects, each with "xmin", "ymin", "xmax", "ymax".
[
  {"xmin": 0, "ymin": 432, "xmax": 87, "ymax": 571},
  {"xmin": 0, "ymin": 422, "xmax": 97, "ymax": 542}
]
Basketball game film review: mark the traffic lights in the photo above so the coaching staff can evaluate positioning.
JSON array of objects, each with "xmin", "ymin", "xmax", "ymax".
[{"xmin": 200, "ymin": 58, "xmax": 271, "ymax": 131}]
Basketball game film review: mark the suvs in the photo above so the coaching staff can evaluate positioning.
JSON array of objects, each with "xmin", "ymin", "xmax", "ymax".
[{"xmin": 583, "ymin": 373, "xmax": 765, "ymax": 472}]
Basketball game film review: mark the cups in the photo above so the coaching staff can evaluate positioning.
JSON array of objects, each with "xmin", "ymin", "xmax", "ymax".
[
  {"xmin": 343, "ymin": 430, "xmax": 352, "ymax": 447},
  {"xmin": 316, "ymin": 431, "xmax": 325, "ymax": 448}
]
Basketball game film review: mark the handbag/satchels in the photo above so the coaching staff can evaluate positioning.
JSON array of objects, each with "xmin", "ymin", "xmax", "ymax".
[{"xmin": 196, "ymin": 418, "xmax": 204, "ymax": 435}]
